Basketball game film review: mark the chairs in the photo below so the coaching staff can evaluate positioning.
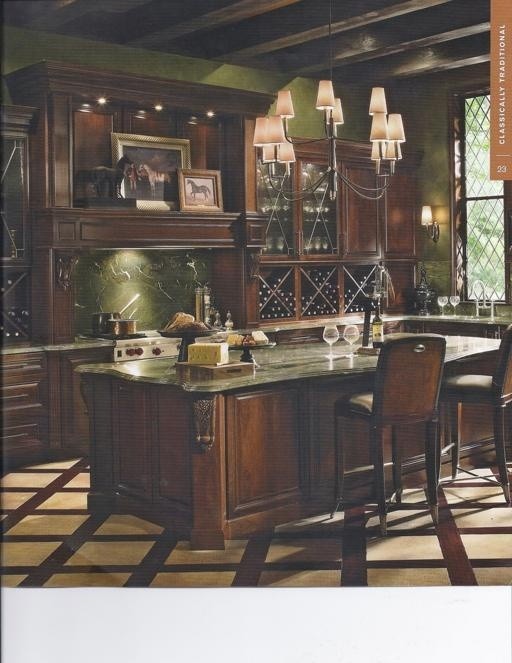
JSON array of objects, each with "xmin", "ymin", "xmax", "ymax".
[
  {"xmin": 442, "ymin": 323, "xmax": 512, "ymax": 506},
  {"xmin": 331, "ymin": 334, "xmax": 447, "ymax": 538}
]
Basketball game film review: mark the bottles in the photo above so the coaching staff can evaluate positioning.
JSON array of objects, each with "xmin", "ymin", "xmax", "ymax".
[{"xmin": 372, "ymin": 306, "xmax": 384, "ymax": 348}]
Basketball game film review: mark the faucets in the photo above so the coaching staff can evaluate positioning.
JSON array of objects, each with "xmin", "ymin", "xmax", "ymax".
[{"xmin": 470, "ymin": 279, "xmax": 494, "ymax": 318}]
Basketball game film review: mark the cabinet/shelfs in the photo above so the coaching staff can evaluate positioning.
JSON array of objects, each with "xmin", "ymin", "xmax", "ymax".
[
  {"xmin": 255, "ymin": 137, "xmax": 343, "ymax": 262},
  {"xmin": 0, "ymin": 352, "xmax": 50, "ymax": 473},
  {"xmin": 5, "ymin": 58, "xmax": 279, "ymax": 214},
  {"xmin": 48, "ymin": 348, "xmax": 114, "ymax": 463},
  {"xmin": 0, "ymin": 104, "xmax": 38, "ymax": 347},
  {"xmin": 270, "ymin": 319, "xmax": 511, "ymax": 338},
  {"xmin": 383, "ymin": 263, "xmax": 421, "ymax": 313},
  {"xmin": 344, "ymin": 141, "xmax": 423, "ymax": 260},
  {"xmin": 31, "ymin": 211, "xmax": 270, "ymax": 344}
]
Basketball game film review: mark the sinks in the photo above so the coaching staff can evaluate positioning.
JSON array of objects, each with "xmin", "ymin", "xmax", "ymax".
[{"xmin": 457, "ymin": 316, "xmax": 500, "ymax": 322}]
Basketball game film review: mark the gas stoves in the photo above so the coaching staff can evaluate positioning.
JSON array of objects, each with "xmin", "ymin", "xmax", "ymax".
[{"xmin": 78, "ymin": 328, "xmax": 183, "ymax": 362}]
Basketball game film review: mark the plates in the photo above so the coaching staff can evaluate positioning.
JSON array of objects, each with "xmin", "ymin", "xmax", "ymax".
[
  {"xmin": 156, "ymin": 329, "xmax": 218, "ymax": 361},
  {"xmin": 228, "ymin": 342, "xmax": 277, "ymax": 368}
]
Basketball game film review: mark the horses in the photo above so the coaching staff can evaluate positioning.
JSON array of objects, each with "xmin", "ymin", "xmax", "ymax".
[
  {"xmin": 90, "ymin": 155, "xmax": 134, "ymax": 200},
  {"xmin": 139, "ymin": 164, "xmax": 169, "ymax": 191},
  {"xmin": 187, "ymin": 178, "xmax": 210, "ymax": 200}
]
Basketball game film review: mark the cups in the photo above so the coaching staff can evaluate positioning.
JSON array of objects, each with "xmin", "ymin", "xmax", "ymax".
[
  {"xmin": 304, "ymin": 235, "xmax": 329, "ymax": 254},
  {"xmin": 303, "ymin": 198, "xmax": 331, "ymax": 222},
  {"xmin": 322, "ymin": 324, "xmax": 339, "ymax": 358},
  {"xmin": 260, "ymin": 197, "xmax": 291, "ymax": 220},
  {"xmin": 343, "ymin": 325, "xmax": 359, "ymax": 358},
  {"xmin": 437, "ymin": 295, "xmax": 447, "ymax": 316},
  {"xmin": 449, "ymin": 295, "xmax": 460, "ymax": 315},
  {"xmin": 262, "ymin": 235, "xmax": 285, "ymax": 254}
]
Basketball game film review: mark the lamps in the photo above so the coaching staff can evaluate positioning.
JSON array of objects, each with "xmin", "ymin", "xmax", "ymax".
[
  {"xmin": 255, "ymin": 3, "xmax": 405, "ymax": 200},
  {"xmin": 420, "ymin": 205, "xmax": 439, "ymax": 243}
]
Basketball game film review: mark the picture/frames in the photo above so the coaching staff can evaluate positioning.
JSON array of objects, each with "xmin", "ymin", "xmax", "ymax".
[
  {"xmin": 110, "ymin": 132, "xmax": 191, "ymax": 210},
  {"xmin": 176, "ymin": 168, "xmax": 224, "ymax": 212}
]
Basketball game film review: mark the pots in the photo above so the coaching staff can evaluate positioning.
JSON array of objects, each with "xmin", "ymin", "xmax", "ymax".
[
  {"xmin": 107, "ymin": 307, "xmax": 139, "ymax": 335},
  {"xmin": 90, "ymin": 293, "xmax": 141, "ymax": 334}
]
